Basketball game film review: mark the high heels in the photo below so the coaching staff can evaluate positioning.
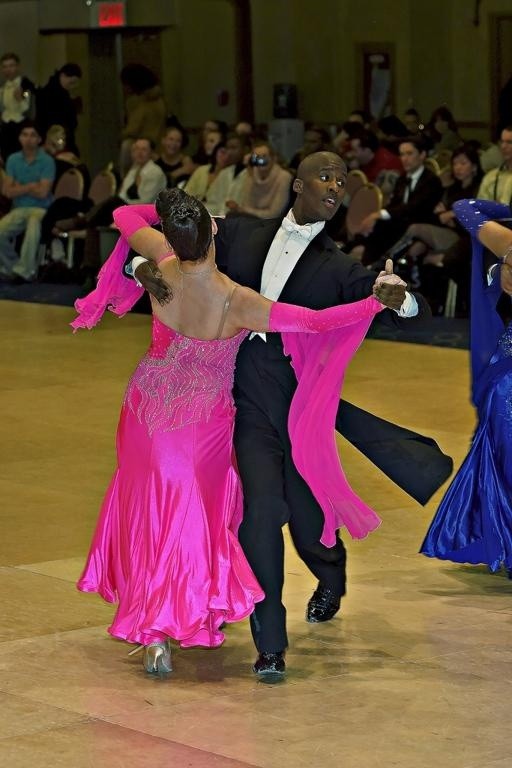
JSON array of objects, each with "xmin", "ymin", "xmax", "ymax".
[{"xmin": 142, "ymin": 636, "xmax": 174, "ymax": 673}]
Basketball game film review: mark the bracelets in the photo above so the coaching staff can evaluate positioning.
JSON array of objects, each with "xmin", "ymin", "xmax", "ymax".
[{"xmin": 503, "ymin": 233, "xmax": 512, "ymax": 266}]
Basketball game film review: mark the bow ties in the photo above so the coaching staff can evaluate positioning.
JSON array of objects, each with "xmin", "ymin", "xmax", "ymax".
[{"xmin": 281, "ymin": 217, "xmax": 312, "ymax": 240}]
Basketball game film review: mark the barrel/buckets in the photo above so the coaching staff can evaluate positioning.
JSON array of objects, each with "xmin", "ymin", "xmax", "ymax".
[{"xmin": 273, "ymin": 83, "xmax": 298, "ymax": 119}]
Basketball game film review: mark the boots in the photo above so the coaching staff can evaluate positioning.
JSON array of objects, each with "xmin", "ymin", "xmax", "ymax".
[{"xmin": 370, "ymin": 232, "xmax": 430, "ymax": 291}]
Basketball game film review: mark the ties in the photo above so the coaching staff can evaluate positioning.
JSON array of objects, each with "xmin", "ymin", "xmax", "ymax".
[{"xmin": 405, "ymin": 177, "xmax": 415, "ymax": 197}]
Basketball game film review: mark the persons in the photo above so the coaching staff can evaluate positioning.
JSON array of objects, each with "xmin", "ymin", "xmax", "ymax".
[
  {"xmin": 417, "ymin": 195, "xmax": 512, "ymax": 586},
  {"xmin": 75, "ymin": 185, "xmax": 408, "ymax": 679},
  {"xmin": 120, "ymin": 149, "xmax": 433, "ymax": 683}
]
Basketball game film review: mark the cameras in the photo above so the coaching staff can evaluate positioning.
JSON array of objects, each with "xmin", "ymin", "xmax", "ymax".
[{"xmin": 249, "ymin": 152, "xmax": 268, "ymax": 167}]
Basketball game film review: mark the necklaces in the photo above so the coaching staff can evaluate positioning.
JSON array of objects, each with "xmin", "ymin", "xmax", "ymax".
[{"xmin": 176, "ymin": 256, "xmax": 219, "ymax": 302}]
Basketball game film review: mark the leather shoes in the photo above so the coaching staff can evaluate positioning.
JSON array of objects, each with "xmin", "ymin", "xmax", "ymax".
[
  {"xmin": 252, "ymin": 652, "xmax": 286, "ymax": 678},
  {"xmin": 305, "ymin": 579, "xmax": 346, "ymax": 624}
]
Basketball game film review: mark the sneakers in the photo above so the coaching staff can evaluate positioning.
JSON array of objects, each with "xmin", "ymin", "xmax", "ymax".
[{"xmin": 0, "ymin": 271, "xmax": 27, "ymax": 286}]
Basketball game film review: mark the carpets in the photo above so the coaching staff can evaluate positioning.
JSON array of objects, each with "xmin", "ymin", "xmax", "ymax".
[{"xmin": 2, "ymin": 270, "xmax": 472, "ymax": 351}]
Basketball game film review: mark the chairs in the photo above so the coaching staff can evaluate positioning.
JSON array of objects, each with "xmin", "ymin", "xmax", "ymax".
[
  {"xmin": 424, "ymin": 158, "xmax": 441, "ymax": 176},
  {"xmin": 34, "ymin": 167, "xmax": 118, "ymax": 269},
  {"xmin": 335, "ymin": 168, "xmax": 384, "ymax": 260}
]
